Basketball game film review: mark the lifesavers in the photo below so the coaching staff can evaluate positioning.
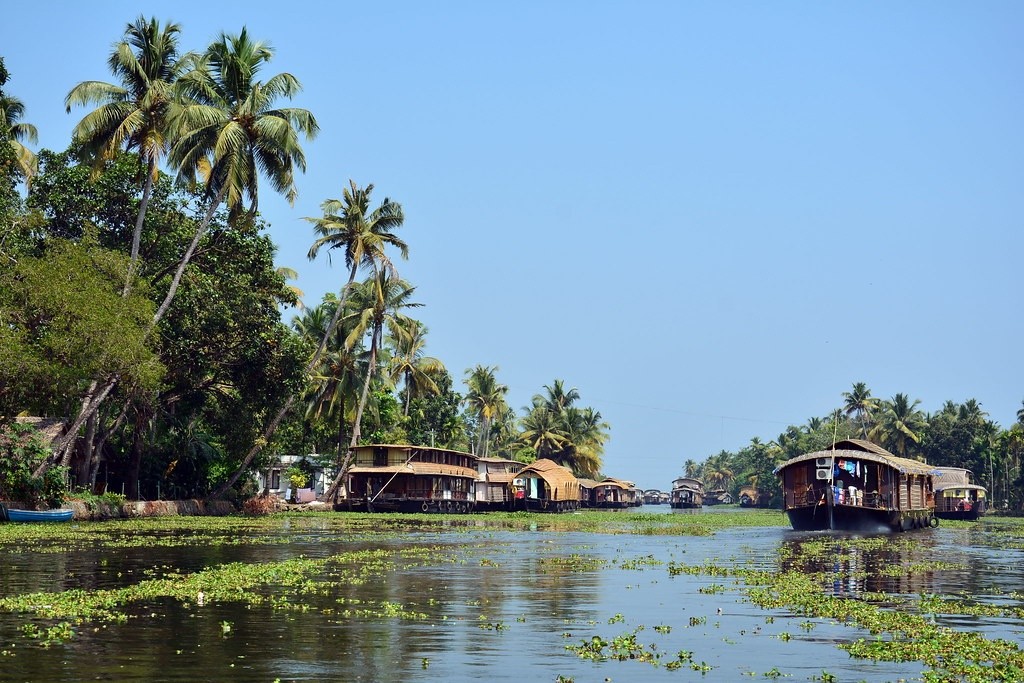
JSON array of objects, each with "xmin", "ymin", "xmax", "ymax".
[
  {"xmin": 462, "ymin": 506, "xmax": 466, "ymax": 512},
  {"xmin": 913, "ymin": 517, "xmax": 919, "ymax": 529},
  {"xmin": 560, "ymin": 502, "xmax": 564, "ymax": 511},
  {"xmin": 422, "ymin": 504, "xmax": 428, "ymax": 511},
  {"xmin": 622, "ymin": 503, "xmax": 631, "ymax": 508},
  {"xmin": 900, "ymin": 520, "xmax": 904, "ymax": 532},
  {"xmin": 929, "ymin": 516, "xmax": 939, "ymax": 528},
  {"xmin": 455, "ymin": 502, "xmax": 460, "ymax": 511},
  {"xmin": 566, "ymin": 502, "xmax": 570, "ymax": 510},
  {"xmin": 926, "ymin": 516, "xmax": 929, "ymax": 527},
  {"xmin": 570, "ymin": 502, "xmax": 574, "ymax": 509},
  {"xmin": 920, "ymin": 516, "xmax": 924, "ymax": 527},
  {"xmin": 438, "ymin": 503, "xmax": 442, "ymax": 510},
  {"xmin": 467, "ymin": 502, "xmax": 474, "ymax": 511},
  {"xmin": 431, "ymin": 505, "xmax": 439, "ymax": 513},
  {"xmin": 446, "ymin": 502, "xmax": 452, "ymax": 510},
  {"xmin": 576, "ymin": 502, "xmax": 579, "ymax": 508}
]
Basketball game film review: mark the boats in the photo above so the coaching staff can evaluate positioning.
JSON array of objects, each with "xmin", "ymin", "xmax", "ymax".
[
  {"xmin": 332, "ymin": 442, "xmax": 482, "ymax": 515},
  {"xmin": 6, "ymin": 508, "xmax": 75, "ymax": 523},
  {"xmin": 668, "ymin": 478, "xmax": 705, "ymax": 509},
  {"xmin": 701, "ymin": 485, "xmax": 765, "ymax": 508},
  {"xmin": 582, "ymin": 476, "xmax": 672, "ymax": 509},
  {"xmin": 771, "ymin": 411, "xmax": 939, "ymax": 540},
  {"xmin": 930, "ymin": 466, "xmax": 989, "ymax": 522},
  {"xmin": 501, "ymin": 457, "xmax": 583, "ymax": 515}
]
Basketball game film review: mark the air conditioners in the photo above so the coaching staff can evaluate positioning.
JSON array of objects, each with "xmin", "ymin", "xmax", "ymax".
[
  {"xmin": 816, "ymin": 458, "xmax": 831, "ymax": 468},
  {"xmin": 816, "ymin": 469, "xmax": 831, "ymax": 480}
]
